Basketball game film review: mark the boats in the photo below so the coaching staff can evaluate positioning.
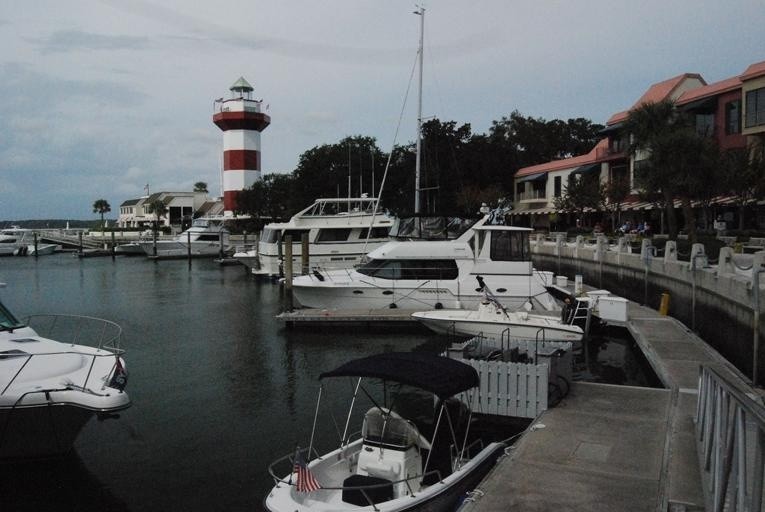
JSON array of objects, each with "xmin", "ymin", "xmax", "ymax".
[
  {"xmin": 0, "ymin": 282, "xmax": 136, "ymax": 465},
  {"xmin": 279, "ymin": 213, "xmax": 575, "ymax": 316},
  {"xmin": 261, "ymin": 352, "xmax": 526, "ymax": 512},
  {"xmin": 411, "ymin": 276, "xmax": 593, "ymax": 348},
  {"xmin": 0, "ymin": 225, "xmax": 58, "ymax": 257},
  {"xmin": 233, "ymin": 192, "xmax": 401, "ymax": 280},
  {"xmin": 115, "ymin": 215, "xmax": 236, "ymax": 257}
]
